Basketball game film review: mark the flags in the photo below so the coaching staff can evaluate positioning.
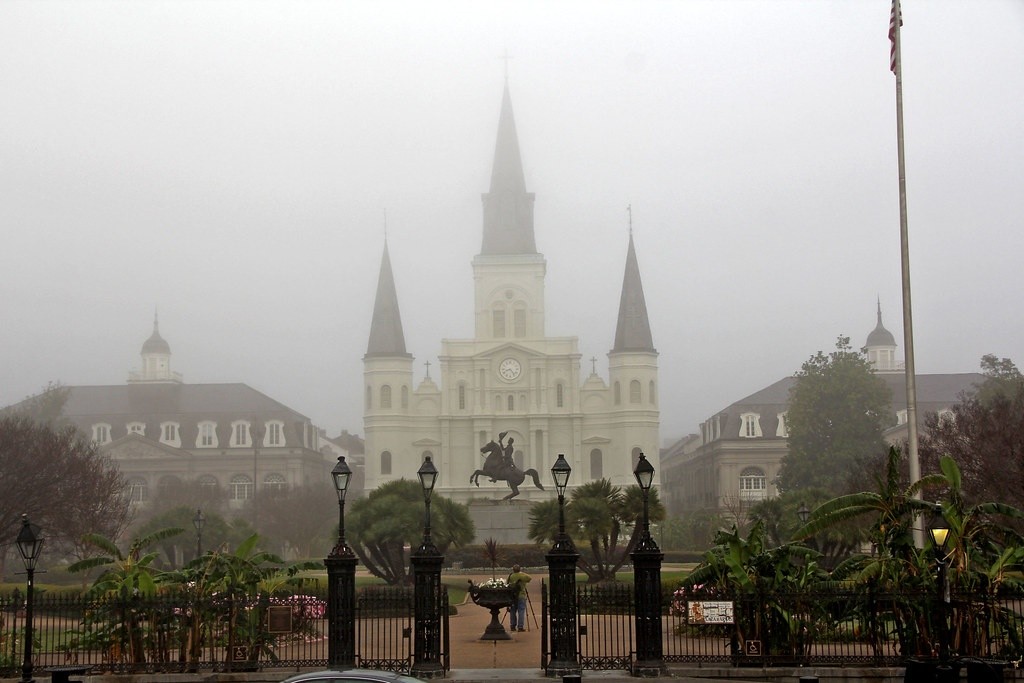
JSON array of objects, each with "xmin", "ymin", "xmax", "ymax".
[{"xmin": 890, "ymin": 0, "xmax": 904, "ymax": 77}]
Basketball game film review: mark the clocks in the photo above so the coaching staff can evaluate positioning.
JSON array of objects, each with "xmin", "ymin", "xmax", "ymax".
[{"xmin": 499, "ymin": 357, "xmax": 521, "ymax": 380}]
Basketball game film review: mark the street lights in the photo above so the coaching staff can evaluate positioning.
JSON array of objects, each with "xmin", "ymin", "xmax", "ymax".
[
  {"xmin": 192, "ymin": 510, "xmax": 206, "ymax": 569},
  {"xmin": 15, "ymin": 514, "xmax": 45, "ymax": 683},
  {"xmin": 549, "ymin": 454, "xmax": 577, "ymax": 554},
  {"xmin": 925, "ymin": 500, "xmax": 953, "ymax": 667},
  {"xmin": 633, "ymin": 452, "xmax": 660, "ymax": 553},
  {"xmin": 327, "ymin": 456, "xmax": 356, "ymax": 558},
  {"xmin": 415, "ymin": 456, "xmax": 442, "ymax": 556}
]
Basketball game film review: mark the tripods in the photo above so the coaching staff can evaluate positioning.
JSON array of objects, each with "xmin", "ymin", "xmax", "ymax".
[{"xmin": 500, "ymin": 588, "xmax": 539, "ymax": 632}]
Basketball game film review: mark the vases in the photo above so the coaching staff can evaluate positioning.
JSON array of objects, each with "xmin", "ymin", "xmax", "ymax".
[{"xmin": 468, "ymin": 579, "xmax": 522, "ymax": 640}]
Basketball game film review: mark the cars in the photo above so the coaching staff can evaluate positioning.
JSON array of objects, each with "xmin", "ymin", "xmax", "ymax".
[{"xmin": 281, "ymin": 668, "xmax": 429, "ymax": 683}]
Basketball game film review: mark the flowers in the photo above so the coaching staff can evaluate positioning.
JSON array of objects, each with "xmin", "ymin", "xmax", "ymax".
[{"xmin": 475, "ymin": 578, "xmax": 509, "ymax": 588}]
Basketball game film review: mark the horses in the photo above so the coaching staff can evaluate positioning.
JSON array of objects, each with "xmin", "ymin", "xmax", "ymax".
[{"xmin": 469, "ymin": 439, "xmax": 545, "ymax": 501}]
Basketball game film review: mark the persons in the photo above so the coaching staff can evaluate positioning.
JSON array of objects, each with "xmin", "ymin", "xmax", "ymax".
[
  {"xmin": 489, "ymin": 438, "xmax": 514, "ymax": 483},
  {"xmin": 506, "ymin": 563, "xmax": 532, "ymax": 631}
]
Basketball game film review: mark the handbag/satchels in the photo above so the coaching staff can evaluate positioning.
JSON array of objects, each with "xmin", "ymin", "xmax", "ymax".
[{"xmin": 509, "ymin": 583, "xmax": 521, "ymax": 601}]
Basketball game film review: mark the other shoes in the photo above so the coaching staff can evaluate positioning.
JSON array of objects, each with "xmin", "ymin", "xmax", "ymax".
[
  {"xmin": 510, "ymin": 626, "xmax": 516, "ymax": 632},
  {"xmin": 517, "ymin": 628, "xmax": 526, "ymax": 632}
]
